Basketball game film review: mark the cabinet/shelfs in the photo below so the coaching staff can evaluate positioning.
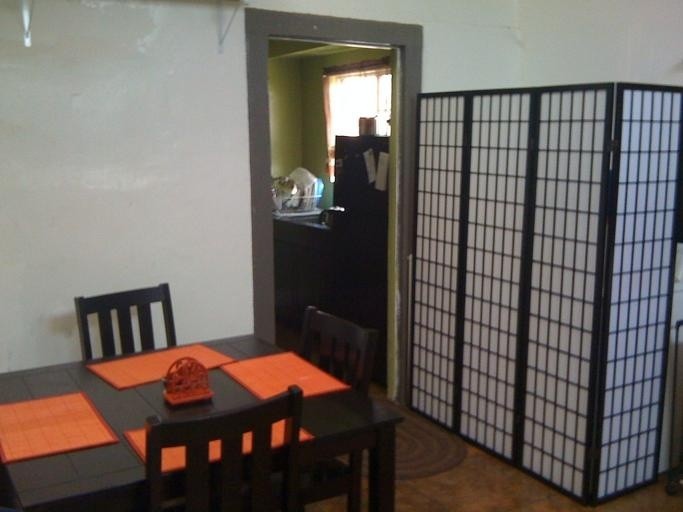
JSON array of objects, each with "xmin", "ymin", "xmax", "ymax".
[{"xmin": 272, "ymin": 217, "xmax": 329, "ymax": 332}]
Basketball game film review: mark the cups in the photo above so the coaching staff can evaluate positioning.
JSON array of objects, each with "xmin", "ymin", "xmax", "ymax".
[{"xmin": 358, "ymin": 117, "xmax": 377, "ymax": 136}]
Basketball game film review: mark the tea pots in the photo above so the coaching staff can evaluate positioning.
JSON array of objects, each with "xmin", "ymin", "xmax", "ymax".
[{"xmin": 316, "ymin": 206, "xmax": 334, "ymax": 227}]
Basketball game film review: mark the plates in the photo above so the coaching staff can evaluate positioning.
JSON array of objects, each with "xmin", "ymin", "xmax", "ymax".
[{"xmin": 271, "ymin": 175, "xmax": 321, "ymax": 215}]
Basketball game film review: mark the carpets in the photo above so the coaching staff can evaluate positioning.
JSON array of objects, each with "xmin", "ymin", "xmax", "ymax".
[{"xmin": 337, "ymin": 395, "xmax": 469, "ymax": 483}]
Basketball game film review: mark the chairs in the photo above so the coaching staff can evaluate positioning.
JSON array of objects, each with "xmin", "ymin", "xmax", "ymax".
[
  {"xmin": 71, "ymin": 280, "xmax": 179, "ymax": 362},
  {"xmin": 141, "ymin": 382, "xmax": 304, "ymax": 511},
  {"xmin": 284, "ymin": 301, "xmax": 382, "ymax": 509}
]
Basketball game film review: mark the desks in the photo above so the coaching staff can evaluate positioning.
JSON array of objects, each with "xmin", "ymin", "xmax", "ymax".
[{"xmin": 0, "ymin": 331, "xmax": 408, "ymax": 511}]
